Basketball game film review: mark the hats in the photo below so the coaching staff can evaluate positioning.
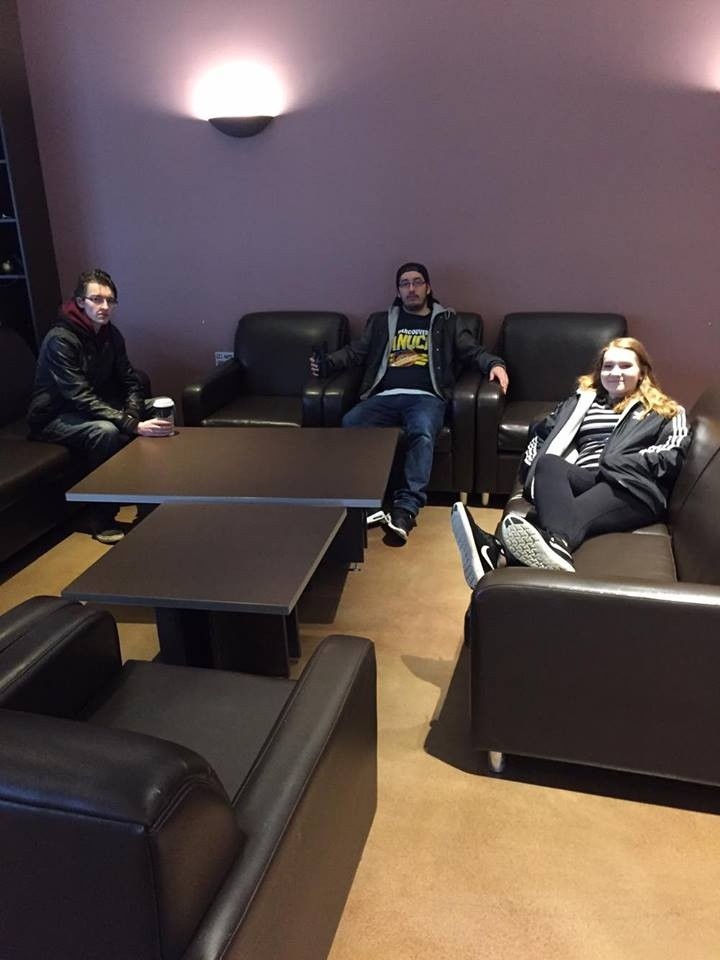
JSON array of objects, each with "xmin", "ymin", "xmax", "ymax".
[{"xmin": 397, "ymin": 263, "xmax": 428, "ymax": 286}]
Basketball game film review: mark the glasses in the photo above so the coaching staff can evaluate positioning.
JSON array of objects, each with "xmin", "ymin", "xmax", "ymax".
[
  {"xmin": 81, "ymin": 295, "xmax": 119, "ymax": 307},
  {"xmin": 398, "ymin": 279, "xmax": 426, "ymax": 289}
]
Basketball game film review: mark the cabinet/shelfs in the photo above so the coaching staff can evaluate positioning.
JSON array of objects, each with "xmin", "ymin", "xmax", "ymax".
[{"xmin": 0, "ymin": 1, "xmax": 65, "ymax": 357}]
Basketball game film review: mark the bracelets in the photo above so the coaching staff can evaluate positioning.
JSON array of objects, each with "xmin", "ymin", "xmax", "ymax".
[{"xmin": 494, "ymin": 363, "xmax": 507, "ymax": 371}]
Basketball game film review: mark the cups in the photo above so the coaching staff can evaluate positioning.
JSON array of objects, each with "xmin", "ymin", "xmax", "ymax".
[{"xmin": 151, "ymin": 397, "xmax": 175, "ymax": 437}]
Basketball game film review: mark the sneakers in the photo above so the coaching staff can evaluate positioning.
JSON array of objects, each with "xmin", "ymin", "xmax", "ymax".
[
  {"xmin": 501, "ymin": 513, "xmax": 577, "ymax": 575},
  {"xmin": 364, "ymin": 507, "xmax": 414, "ymax": 541},
  {"xmin": 89, "ymin": 517, "xmax": 124, "ymax": 543},
  {"xmin": 451, "ymin": 501, "xmax": 501, "ymax": 592}
]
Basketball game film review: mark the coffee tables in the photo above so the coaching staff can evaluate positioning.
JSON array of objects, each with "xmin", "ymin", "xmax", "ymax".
[
  {"xmin": 62, "ymin": 497, "xmax": 350, "ymax": 676},
  {"xmin": 64, "ymin": 427, "xmax": 400, "ymax": 564}
]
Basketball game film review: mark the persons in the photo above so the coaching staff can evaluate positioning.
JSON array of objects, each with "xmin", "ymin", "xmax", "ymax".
[
  {"xmin": 22, "ymin": 270, "xmax": 177, "ymax": 547},
  {"xmin": 309, "ymin": 261, "xmax": 509, "ymax": 545},
  {"xmin": 448, "ymin": 336, "xmax": 692, "ymax": 594}
]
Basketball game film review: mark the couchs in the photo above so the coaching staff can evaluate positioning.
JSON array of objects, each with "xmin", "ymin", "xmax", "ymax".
[
  {"xmin": 1, "ymin": 329, "xmax": 154, "ymax": 567},
  {"xmin": 463, "ymin": 382, "xmax": 720, "ymax": 791},
  {"xmin": 181, "ymin": 309, "xmax": 348, "ymax": 430},
  {"xmin": 0, "ymin": 604, "xmax": 378, "ymax": 958},
  {"xmin": 479, "ymin": 312, "xmax": 630, "ymax": 510},
  {"xmin": 326, "ymin": 310, "xmax": 485, "ymax": 506}
]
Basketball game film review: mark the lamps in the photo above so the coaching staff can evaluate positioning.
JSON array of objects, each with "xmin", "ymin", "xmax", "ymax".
[{"xmin": 207, "ymin": 116, "xmax": 272, "ymax": 139}]
